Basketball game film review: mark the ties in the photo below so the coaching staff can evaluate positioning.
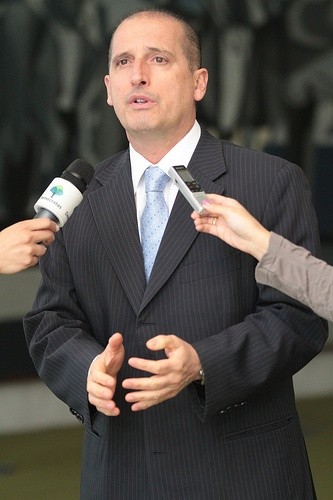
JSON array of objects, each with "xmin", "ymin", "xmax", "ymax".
[{"xmin": 139, "ymin": 166, "xmax": 172, "ymax": 284}]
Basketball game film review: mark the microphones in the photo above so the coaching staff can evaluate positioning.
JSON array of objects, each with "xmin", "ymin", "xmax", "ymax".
[{"xmin": 32, "ymin": 160, "xmax": 95, "ymax": 245}]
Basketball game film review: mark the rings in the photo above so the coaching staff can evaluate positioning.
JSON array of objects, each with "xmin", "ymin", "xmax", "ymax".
[{"xmin": 213, "ymin": 216, "xmax": 217, "ymax": 225}]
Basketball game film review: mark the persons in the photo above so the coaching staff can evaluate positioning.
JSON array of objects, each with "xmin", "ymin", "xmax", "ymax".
[
  {"xmin": 192, "ymin": 193, "xmax": 333, "ymax": 328},
  {"xmin": 0, "ymin": 219, "xmax": 57, "ymax": 275},
  {"xmin": 21, "ymin": 9, "xmax": 329, "ymax": 500}
]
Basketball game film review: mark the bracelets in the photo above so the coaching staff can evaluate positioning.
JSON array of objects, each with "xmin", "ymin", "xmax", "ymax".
[{"xmin": 198, "ymin": 368, "xmax": 204, "ymax": 382}]
